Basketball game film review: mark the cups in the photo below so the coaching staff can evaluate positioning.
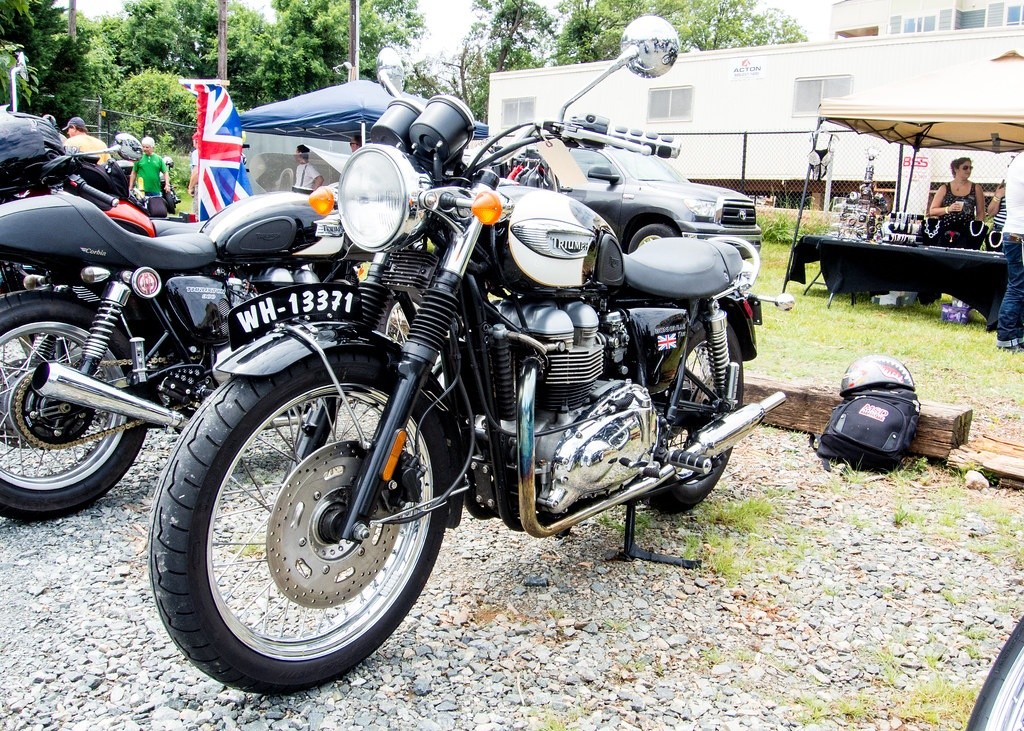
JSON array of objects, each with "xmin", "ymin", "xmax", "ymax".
[{"xmin": 955, "ymin": 201, "xmax": 965, "ymax": 211}]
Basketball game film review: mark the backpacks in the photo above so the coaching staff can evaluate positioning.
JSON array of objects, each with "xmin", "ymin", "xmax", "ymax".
[{"xmin": 818, "ymin": 386, "xmax": 921, "ymax": 471}]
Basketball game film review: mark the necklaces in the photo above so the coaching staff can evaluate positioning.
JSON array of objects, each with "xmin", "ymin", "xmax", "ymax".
[
  {"xmin": 970, "ymin": 221, "xmax": 984, "ymax": 236},
  {"xmin": 925, "ymin": 219, "xmax": 940, "ymax": 238},
  {"xmin": 989, "ymin": 231, "xmax": 1003, "ymax": 248},
  {"xmin": 954, "ymin": 180, "xmax": 968, "ymax": 191}
]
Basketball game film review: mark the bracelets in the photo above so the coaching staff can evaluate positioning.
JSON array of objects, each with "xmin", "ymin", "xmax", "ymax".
[
  {"xmin": 992, "ymin": 196, "xmax": 1001, "ymax": 203},
  {"xmin": 945, "ymin": 207, "xmax": 950, "ymax": 214}
]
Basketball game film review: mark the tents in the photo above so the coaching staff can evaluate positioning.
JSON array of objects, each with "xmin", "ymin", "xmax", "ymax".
[
  {"xmin": 782, "ymin": 50, "xmax": 1024, "ymax": 294},
  {"xmin": 238, "ymin": 79, "xmax": 430, "ymax": 147}
]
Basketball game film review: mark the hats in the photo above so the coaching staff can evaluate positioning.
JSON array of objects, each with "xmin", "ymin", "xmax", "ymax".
[
  {"xmin": 62, "ymin": 117, "xmax": 87, "ymax": 132},
  {"xmin": 191, "ymin": 133, "xmax": 197, "ymax": 138}
]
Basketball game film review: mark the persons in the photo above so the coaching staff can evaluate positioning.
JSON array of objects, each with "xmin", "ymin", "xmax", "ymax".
[
  {"xmin": 987, "ymin": 186, "xmax": 1006, "ymax": 231},
  {"xmin": 295, "ymin": 145, "xmax": 324, "ymax": 191},
  {"xmin": 61, "ymin": 117, "xmax": 111, "ymax": 165},
  {"xmin": 929, "ymin": 157, "xmax": 985, "ymax": 222},
  {"xmin": 46, "ymin": 115, "xmax": 66, "ymax": 140},
  {"xmin": 996, "ymin": 151, "xmax": 1024, "ymax": 351},
  {"xmin": 188, "ymin": 131, "xmax": 198, "ymax": 215},
  {"xmin": 129, "ymin": 137, "xmax": 170, "ymax": 197},
  {"xmin": 351, "ymin": 135, "xmax": 362, "ymax": 152}
]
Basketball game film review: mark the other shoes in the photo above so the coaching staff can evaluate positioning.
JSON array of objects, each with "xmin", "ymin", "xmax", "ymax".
[{"xmin": 999, "ymin": 344, "xmax": 1024, "ymax": 352}]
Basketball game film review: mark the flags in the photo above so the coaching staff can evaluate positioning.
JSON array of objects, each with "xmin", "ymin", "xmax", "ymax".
[{"xmin": 182, "ymin": 79, "xmax": 252, "ymax": 223}]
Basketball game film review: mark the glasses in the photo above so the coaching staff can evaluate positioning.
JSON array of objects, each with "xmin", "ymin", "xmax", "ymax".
[
  {"xmin": 958, "ymin": 166, "xmax": 974, "ymax": 170},
  {"xmin": 349, "ymin": 142, "xmax": 357, "ymax": 146}
]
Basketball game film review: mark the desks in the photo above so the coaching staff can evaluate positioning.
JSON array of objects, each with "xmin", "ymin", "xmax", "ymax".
[{"xmin": 786, "ymin": 234, "xmax": 1008, "ymax": 333}]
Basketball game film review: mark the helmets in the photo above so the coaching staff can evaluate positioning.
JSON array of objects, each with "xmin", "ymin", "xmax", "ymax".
[{"xmin": 840, "ymin": 355, "xmax": 914, "ymax": 393}]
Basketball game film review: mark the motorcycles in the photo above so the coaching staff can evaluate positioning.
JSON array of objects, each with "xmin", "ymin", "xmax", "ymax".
[
  {"xmin": 142, "ymin": 13, "xmax": 786, "ymax": 693},
  {"xmin": 0, "ymin": 99, "xmax": 211, "ymax": 237},
  {"xmin": 0, "ymin": 192, "xmax": 351, "ymax": 516}
]
